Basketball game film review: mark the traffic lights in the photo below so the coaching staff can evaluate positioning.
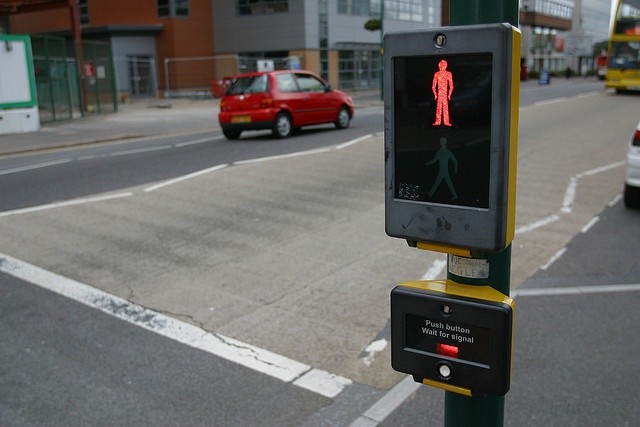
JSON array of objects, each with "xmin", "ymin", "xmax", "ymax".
[{"xmin": 384, "ymin": 22, "xmax": 522, "ymax": 256}]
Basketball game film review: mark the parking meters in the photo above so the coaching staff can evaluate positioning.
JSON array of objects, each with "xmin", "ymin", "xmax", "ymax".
[{"xmin": 391, "ymin": 279, "xmax": 516, "ymax": 397}]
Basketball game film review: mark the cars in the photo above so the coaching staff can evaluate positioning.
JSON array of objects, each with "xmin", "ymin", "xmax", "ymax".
[
  {"xmin": 219, "ymin": 69, "xmax": 355, "ymax": 138},
  {"xmin": 624, "ymin": 119, "xmax": 640, "ymax": 211}
]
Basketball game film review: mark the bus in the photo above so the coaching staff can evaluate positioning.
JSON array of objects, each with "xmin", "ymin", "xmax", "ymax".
[{"xmin": 603, "ymin": 16, "xmax": 640, "ymax": 94}]
